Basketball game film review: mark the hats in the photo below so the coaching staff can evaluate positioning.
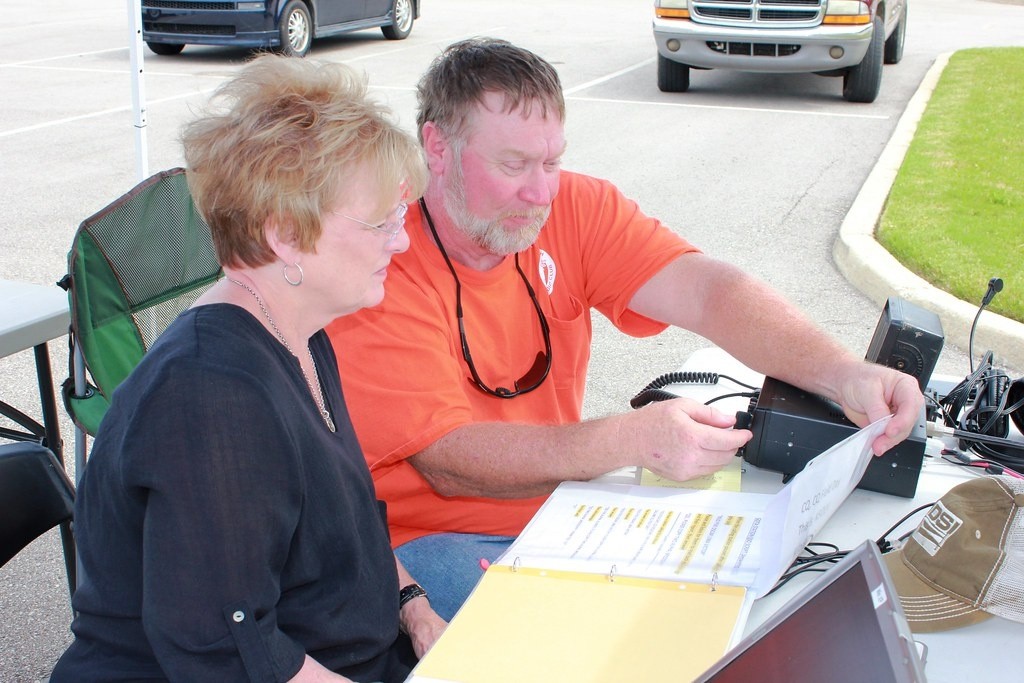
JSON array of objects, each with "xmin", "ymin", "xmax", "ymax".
[{"xmin": 882, "ymin": 475, "xmax": 1024, "ymax": 634}]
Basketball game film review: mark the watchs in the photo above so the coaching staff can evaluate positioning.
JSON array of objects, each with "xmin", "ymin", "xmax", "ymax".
[{"xmin": 398, "ymin": 583, "xmax": 427, "ymax": 610}]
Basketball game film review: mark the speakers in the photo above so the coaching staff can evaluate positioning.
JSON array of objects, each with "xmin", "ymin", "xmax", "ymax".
[{"xmin": 864, "ymin": 298, "xmax": 945, "ymax": 394}]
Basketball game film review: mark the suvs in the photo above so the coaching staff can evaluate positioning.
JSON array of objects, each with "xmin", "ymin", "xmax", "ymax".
[{"xmin": 652, "ymin": 0, "xmax": 907, "ymax": 103}]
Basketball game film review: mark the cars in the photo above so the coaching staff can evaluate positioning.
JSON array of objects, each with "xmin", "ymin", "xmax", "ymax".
[{"xmin": 142, "ymin": 0, "xmax": 422, "ymax": 60}]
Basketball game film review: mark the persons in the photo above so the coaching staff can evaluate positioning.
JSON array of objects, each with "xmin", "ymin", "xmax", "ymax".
[
  {"xmin": 324, "ymin": 38, "xmax": 921, "ymax": 623},
  {"xmin": 48, "ymin": 55, "xmax": 449, "ymax": 683}
]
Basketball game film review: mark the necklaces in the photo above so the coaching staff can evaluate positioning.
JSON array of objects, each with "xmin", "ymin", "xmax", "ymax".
[{"xmin": 222, "ymin": 277, "xmax": 337, "ymax": 434}]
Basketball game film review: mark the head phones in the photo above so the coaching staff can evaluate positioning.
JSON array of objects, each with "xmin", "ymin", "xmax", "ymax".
[{"xmin": 960, "ymin": 367, "xmax": 1024, "ymax": 473}]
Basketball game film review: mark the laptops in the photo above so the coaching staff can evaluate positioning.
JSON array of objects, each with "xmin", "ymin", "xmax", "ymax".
[{"xmin": 690, "ymin": 538, "xmax": 925, "ymax": 683}]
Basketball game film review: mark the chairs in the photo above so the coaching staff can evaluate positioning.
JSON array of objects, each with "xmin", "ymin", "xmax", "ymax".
[
  {"xmin": 58, "ymin": 177, "xmax": 234, "ymax": 434},
  {"xmin": 1, "ymin": 441, "xmax": 84, "ymax": 631}
]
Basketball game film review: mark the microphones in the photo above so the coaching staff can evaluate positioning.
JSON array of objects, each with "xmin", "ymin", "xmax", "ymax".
[{"xmin": 969, "ymin": 277, "xmax": 1004, "ymax": 374}]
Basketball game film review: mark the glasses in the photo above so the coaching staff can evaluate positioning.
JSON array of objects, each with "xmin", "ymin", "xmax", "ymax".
[
  {"xmin": 327, "ymin": 202, "xmax": 408, "ymax": 249},
  {"xmin": 457, "ymin": 294, "xmax": 552, "ymax": 398}
]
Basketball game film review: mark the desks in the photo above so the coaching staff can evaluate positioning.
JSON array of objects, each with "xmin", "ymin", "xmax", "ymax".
[
  {"xmin": 0, "ymin": 281, "xmax": 91, "ymax": 561},
  {"xmin": 402, "ymin": 345, "xmax": 1023, "ymax": 683}
]
